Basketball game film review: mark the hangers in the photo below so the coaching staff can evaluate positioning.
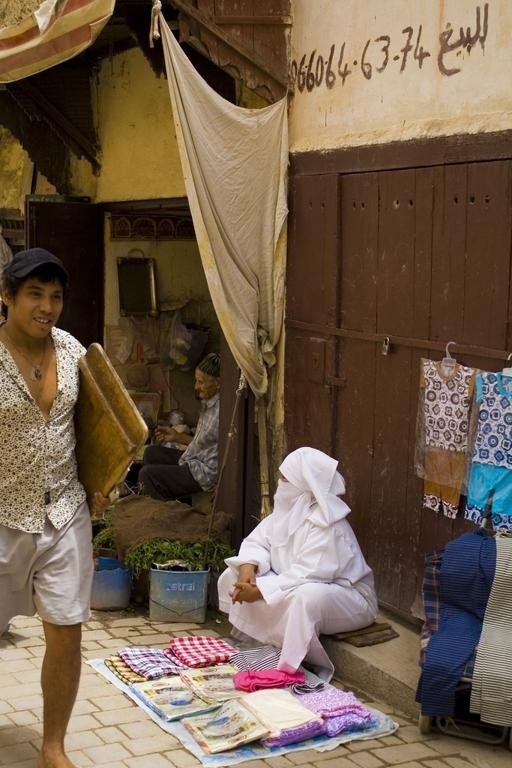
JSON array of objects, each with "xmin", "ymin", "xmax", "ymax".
[
  {"xmin": 440, "ymin": 341, "xmax": 457, "ymax": 366},
  {"xmin": 501, "ymin": 354, "xmax": 512, "ymax": 378}
]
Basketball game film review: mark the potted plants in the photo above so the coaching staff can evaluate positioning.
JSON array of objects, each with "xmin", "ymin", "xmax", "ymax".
[{"xmin": 125, "ymin": 538, "xmax": 237, "ymax": 623}]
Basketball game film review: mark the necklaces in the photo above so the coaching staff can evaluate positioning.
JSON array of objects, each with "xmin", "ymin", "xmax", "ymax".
[{"xmin": 3, "ymin": 325, "xmax": 47, "ymax": 382}]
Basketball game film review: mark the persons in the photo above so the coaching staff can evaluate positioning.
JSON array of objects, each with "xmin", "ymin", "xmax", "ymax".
[
  {"xmin": 216, "ymin": 447, "xmax": 379, "ymax": 672},
  {"xmin": 138, "ymin": 354, "xmax": 220, "ymax": 500},
  {"xmin": 0, "ymin": 248, "xmax": 113, "ymax": 768}
]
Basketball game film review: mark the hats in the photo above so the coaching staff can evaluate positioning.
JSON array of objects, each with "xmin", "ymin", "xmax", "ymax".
[
  {"xmin": 198, "ymin": 351, "xmax": 222, "ymax": 377},
  {"xmin": 4, "ymin": 246, "xmax": 69, "ymax": 283}
]
